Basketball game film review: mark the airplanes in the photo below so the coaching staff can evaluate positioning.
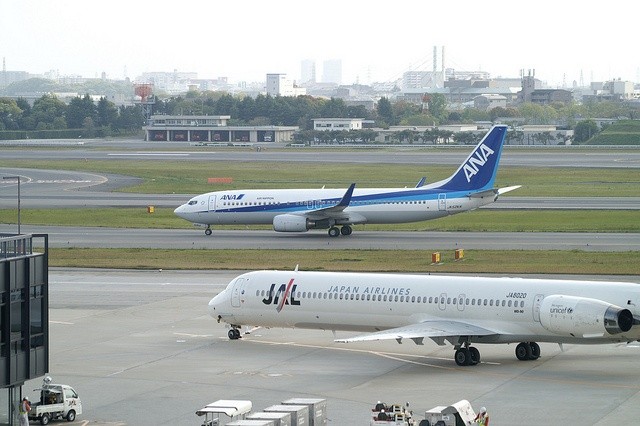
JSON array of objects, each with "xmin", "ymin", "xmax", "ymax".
[
  {"xmin": 209, "ymin": 264, "xmax": 640, "ymax": 366},
  {"xmin": 174, "ymin": 125, "xmax": 522, "ymax": 237}
]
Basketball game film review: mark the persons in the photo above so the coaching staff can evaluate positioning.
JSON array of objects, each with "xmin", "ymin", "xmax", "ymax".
[{"xmin": 19, "ymin": 396, "xmax": 31, "ymax": 425}]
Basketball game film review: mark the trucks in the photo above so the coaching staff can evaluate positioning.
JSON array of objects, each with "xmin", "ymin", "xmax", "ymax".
[{"xmin": 28, "ymin": 384, "xmax": 82, "ymax": 425}]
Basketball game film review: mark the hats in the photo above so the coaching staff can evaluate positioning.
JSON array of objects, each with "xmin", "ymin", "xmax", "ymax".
[{"xmin": 480, "ymin": 407, "xmax": 486, "ymax": 414}]
